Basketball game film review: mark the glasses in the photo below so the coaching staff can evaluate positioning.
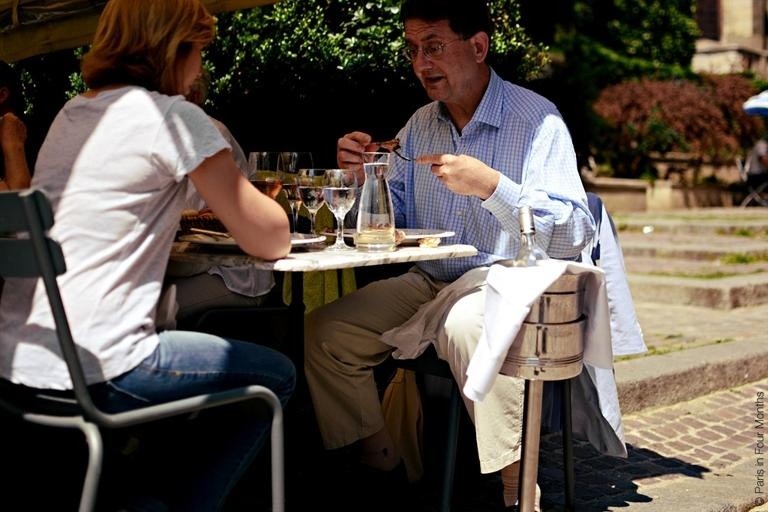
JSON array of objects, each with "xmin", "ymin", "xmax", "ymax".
[{"xmin": 404, "ymin": 38, "xmax": 463, "ymax": 63}]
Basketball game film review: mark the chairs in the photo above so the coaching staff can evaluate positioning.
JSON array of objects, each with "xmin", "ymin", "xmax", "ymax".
[
  {"xmin": 366, "ymin": 193, "xmax": 605, "ymax": 512},
  {"xmin": 0, "ymin": 181, "xmax": 290, "ymax": 512},
  {"xmin": 733, "ymin": 154, "xmax": 767, "ymax": 206}
]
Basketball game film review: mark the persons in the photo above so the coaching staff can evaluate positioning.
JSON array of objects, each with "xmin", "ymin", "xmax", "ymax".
[
  {"xmin": 744, "ymin": 132, "xmax": 768, "ymax": 199},
  {"xmin": 1, "ymin": 55, "xmax": 32, "ymax": 191},
  {"xmin": 305, "ymin": 0, "xmax": 597, "ymax": 511},
  {"xmin": 0, "ymin": 0, "xmax": 296, "ymax": 512}
]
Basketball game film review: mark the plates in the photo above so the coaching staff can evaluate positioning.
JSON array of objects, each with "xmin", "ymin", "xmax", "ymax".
[
  {"xmin": 325, "ymin": 225, "xmax": 456, "ymax": 244},
  {"xmin": 180, "ymin": 231, "xmax": 326, "ymax": 249}
]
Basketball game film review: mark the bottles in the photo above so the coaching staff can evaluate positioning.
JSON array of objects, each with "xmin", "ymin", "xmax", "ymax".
[
  {"xmin": 516, "ymin": 200, "xmax": 551, "ymax": 266},
  {"xmin": 355, "ymin": 151, "xmax": 401, "ymax": 253}
]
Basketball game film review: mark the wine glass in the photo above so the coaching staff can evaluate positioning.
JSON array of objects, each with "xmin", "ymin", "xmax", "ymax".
[
  {"xmin": 247, "ymin": 150, "xmax": 323, "ymax": 237},
  {"xmin": 321, "ymin": 169, "xmax": 355, "ymax": 249}
]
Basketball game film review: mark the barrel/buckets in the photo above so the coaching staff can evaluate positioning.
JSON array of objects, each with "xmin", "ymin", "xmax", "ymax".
[{"xmin": 490, "ymin": 258, "xmax": 588, "ymax": 380}]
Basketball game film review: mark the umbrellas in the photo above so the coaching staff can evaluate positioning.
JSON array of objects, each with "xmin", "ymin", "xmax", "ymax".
[{"xmin": 744, "ymin": 90, "xmax": 768, "ymax": 115}]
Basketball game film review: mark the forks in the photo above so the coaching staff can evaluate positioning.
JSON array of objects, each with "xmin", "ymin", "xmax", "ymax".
[{"xmin": 391, "ymin": 145, "xmax": 441, "ymax": 167}]
